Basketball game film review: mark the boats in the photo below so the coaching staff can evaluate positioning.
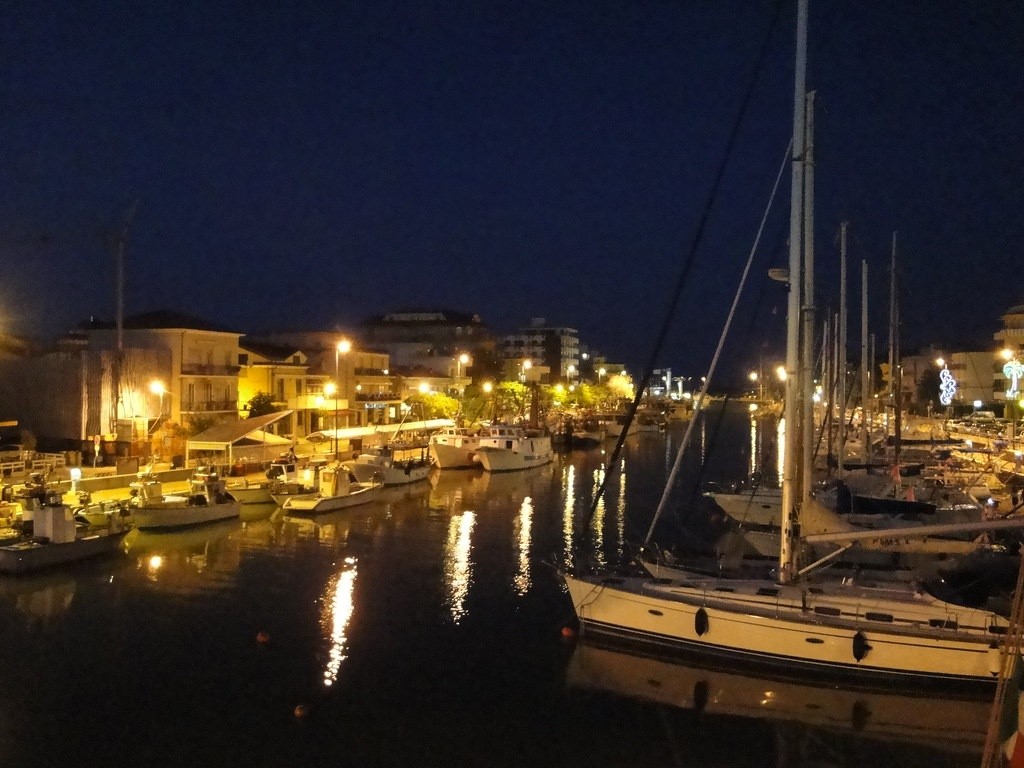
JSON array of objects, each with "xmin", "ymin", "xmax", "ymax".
[
  {"xmin": 428, "ymin": 373, "xmax": 554, "ymax": 471},
  {"xmin": 0, "ymin": 463, "xmax": 136, "ymax": 573},
  {"xmin": 78, "ymin": 455, "xmax": 245, "ymax": 528},
  {"xmin": 225, "ymin": 403, "xmax": 438, "ymax": 512},
  {"xmin": 549, "ymin": 388, "xmax": 667, "ymax": 442}
]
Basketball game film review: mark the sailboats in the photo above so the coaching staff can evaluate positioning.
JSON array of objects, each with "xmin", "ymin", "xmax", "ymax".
[{"xmin": 564, "ymin": 0, "xmax": 1024, "ymax": 684}]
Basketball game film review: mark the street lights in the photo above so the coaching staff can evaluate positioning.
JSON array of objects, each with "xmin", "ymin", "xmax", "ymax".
[
  {"xmin": 336, "ymin": 340, "xmax": 351, "ymax": 460},
  {"xmin": 567, "ymin": 365, "xmax": 626, "ymax": 385},
  {"xmin": 151, "ymin": 382, "xmax": 163, "ymax": 427},
  {"xmin": 458, "ymin": 354, "xmax": 469, "ymax": 378},
  {"xmin": 522, "ymin": 360, "xmax": 531, "ymax": 418}
]
